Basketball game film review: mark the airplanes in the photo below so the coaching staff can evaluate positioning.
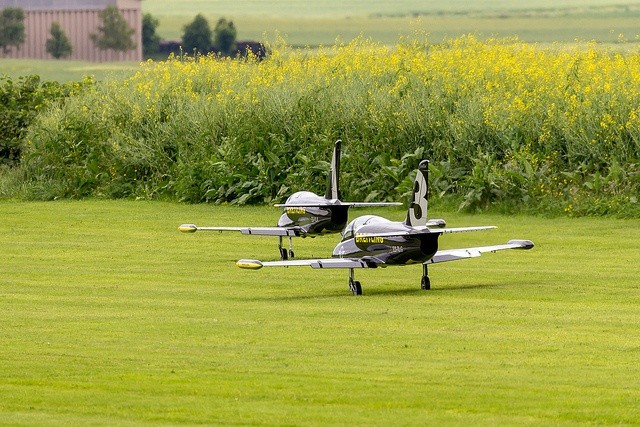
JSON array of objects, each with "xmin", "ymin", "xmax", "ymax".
[
  {"xmin": 234, "ymin": 160, "xmax": 535, "ymax": 295},
  {"xmin": 177, "ymin": 140, "xmax": 405, "ymax": 260}
]
